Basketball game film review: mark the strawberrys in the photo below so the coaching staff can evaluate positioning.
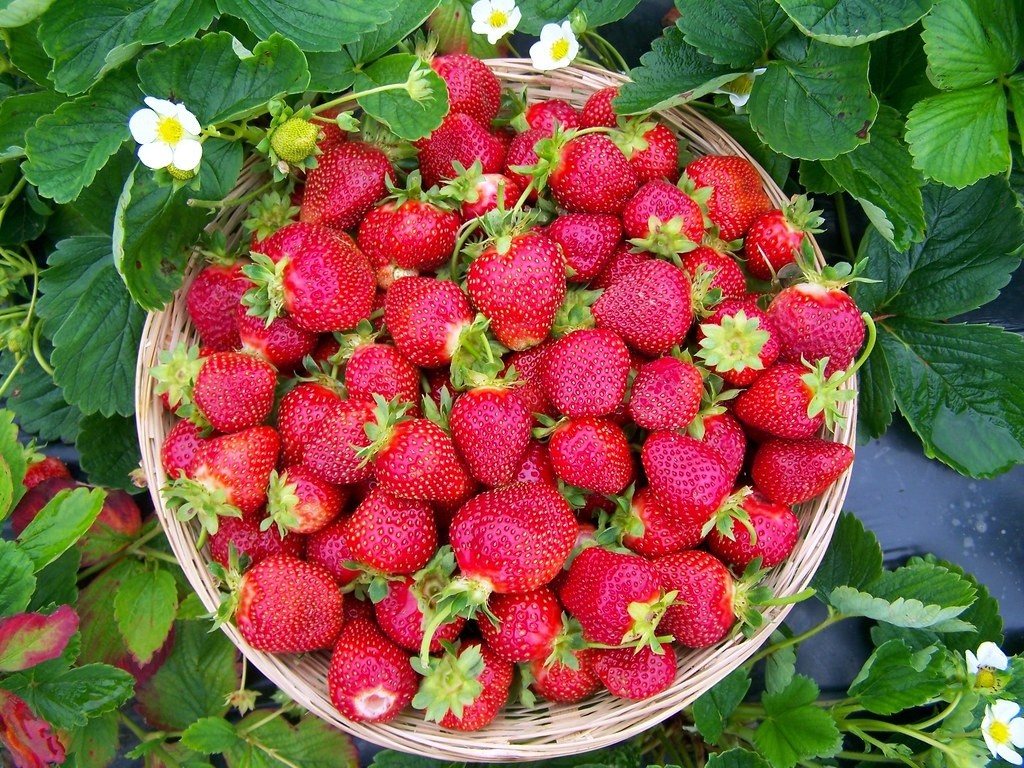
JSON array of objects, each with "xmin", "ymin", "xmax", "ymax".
[
  {"xmin": 23, "ymin": 457, "xmax": 71, "ymax": 489},
  {"xmin": 152, "ymin": 52, "xmax": 867, "ymax": 730}
]
